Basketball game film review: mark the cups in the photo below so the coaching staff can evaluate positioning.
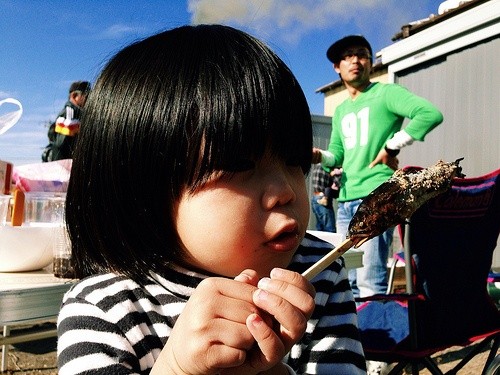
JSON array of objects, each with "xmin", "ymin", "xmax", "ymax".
[{"xmin": 0, "ymin": 190, "xmax": 71, "ymax": 273}]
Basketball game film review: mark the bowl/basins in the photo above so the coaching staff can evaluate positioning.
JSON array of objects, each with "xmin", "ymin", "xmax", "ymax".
[{"xmin": 0, "ymin": 222, "xmax": 66, "ymax": 273}]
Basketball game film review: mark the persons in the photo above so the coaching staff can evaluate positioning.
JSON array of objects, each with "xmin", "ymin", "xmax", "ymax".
[
  {"xmin": 48, "ymin": 80, "xmax": 90, "ymax": 186},
  {"xmin": 57, "ymin": 23, "xmax": 368, "ymax": 375},
  {"xmin": 310, "ymin": 162, "xmax": 341, "ymax": 233},
  {"xmin": 311, "ymin": 34, "xmax": 444, "ymax": 375}
]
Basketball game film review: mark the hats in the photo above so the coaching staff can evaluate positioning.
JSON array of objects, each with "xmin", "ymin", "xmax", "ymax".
[
  {"xmin": 325, "ymin": 33, "xmax": 374, "ymax": 62},
  {"xmin": 69, "ymin": 81, "xmax": 91, "ymax": 93}
]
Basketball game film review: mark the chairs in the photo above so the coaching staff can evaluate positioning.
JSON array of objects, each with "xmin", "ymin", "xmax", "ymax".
[{"xmin": 357, "ymin": 167, "xmax": 500, "ymax": 375}]
